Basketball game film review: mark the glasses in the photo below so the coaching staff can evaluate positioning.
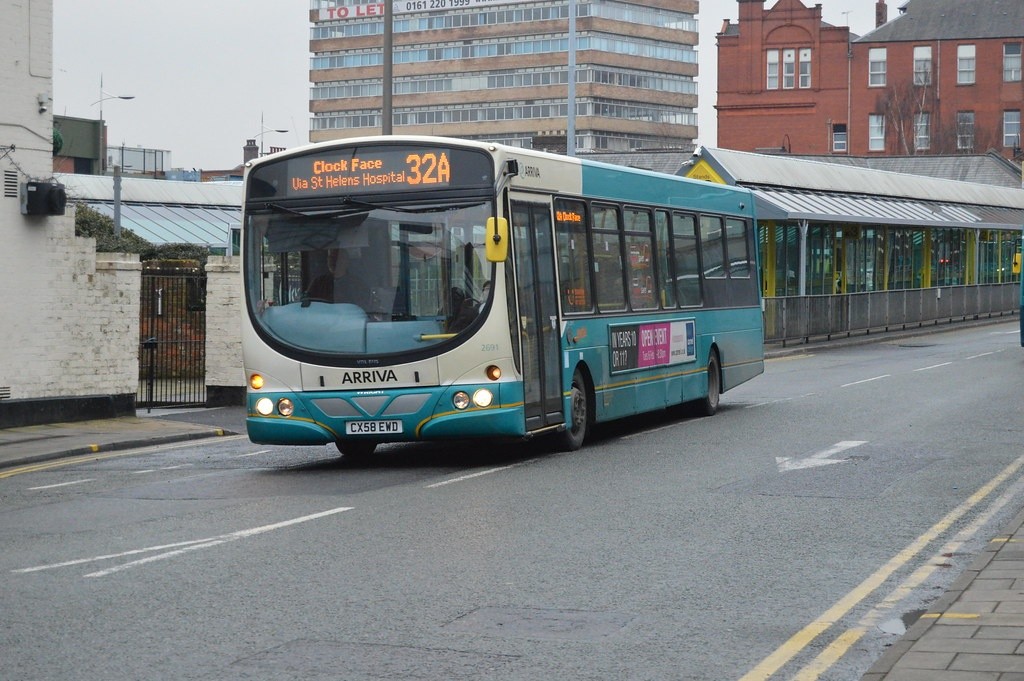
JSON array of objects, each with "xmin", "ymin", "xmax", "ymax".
[{"xmin": 327, "ymin": 256, "xmax": 343, "ymax": 262}]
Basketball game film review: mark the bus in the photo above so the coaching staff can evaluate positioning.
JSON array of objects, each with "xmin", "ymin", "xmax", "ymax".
[{"xmin": 235, "ymin": 136, "xmax": 768, "ymax": 464}]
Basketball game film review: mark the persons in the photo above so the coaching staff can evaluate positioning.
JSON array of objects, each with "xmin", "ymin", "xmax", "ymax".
[
  {"xmin": 302, "ymin": 248, "xmax": 370, "ymax": 312},
  {"xmin": 436, "ymin": 280, "xmax": 491, "ymax": 333}
]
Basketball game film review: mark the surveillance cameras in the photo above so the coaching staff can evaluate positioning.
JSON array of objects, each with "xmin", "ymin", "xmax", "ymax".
[{"xmin": 693, "ymin": 147, "xmax": 701, "ymax": 157}]
